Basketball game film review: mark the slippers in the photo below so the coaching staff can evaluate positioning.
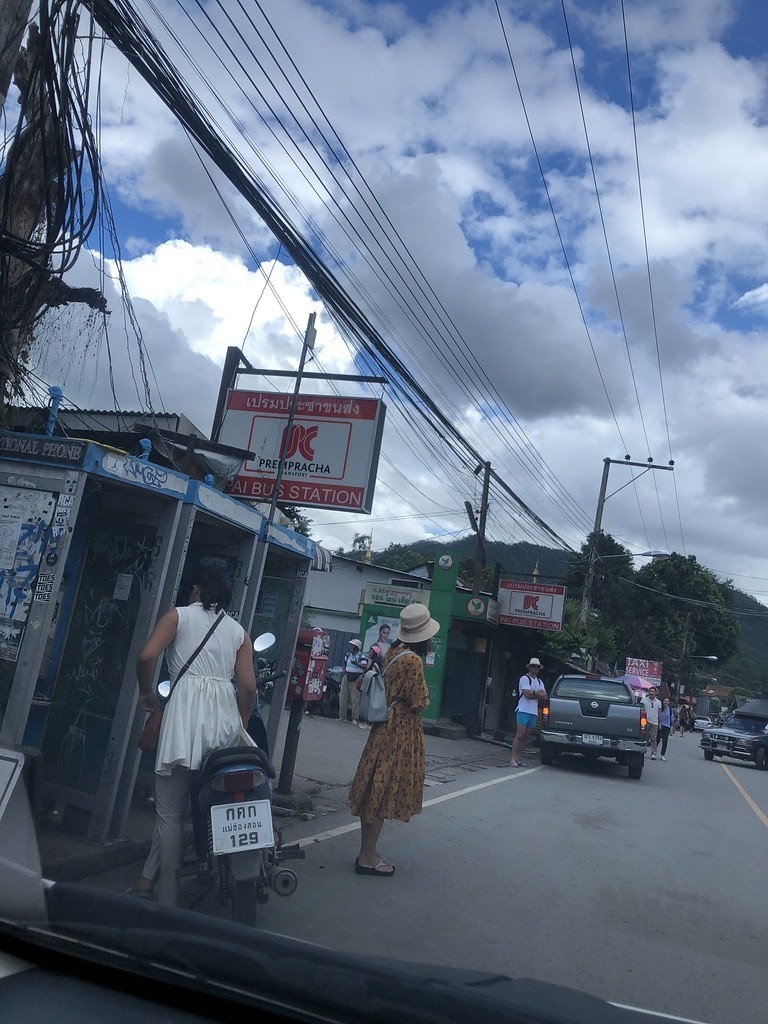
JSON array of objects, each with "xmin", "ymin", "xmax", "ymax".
[
  {"xmin": 124, "ymin": 882, "xmax": 155, "ymax": 900},
  {"xmin": 354, "ymin": 853, "xmax": 395, "ymax": 876}
]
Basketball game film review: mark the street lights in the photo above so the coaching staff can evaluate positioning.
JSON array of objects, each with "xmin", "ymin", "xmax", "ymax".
[{"xmin": 576, "ymin": 550, "xmax": 670, "ymax": 666}]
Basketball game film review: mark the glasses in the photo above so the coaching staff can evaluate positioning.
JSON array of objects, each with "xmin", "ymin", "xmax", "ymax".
[
  {"xmin": 531, "ymin": 665, "xmax": 541, "ymax": 670},
  {"xmin": 651, "ymin": 703, "xmax": 653, "ymax": 708}
]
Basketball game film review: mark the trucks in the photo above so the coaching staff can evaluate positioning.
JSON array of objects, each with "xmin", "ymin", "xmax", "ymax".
[{"xmin": 693, "ymin": 715, "xmax": 711, "ymax": 731}]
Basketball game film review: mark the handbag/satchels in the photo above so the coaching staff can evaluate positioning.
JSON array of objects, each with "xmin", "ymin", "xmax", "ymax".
[
  {"xmin": 135, "ymin": 705, "xmax": 164, "ymax": 752},
  {"xmin": 356, "ymin": 679, "xmax": 362, "ymax": 691},
  {"xmin": 346, "ymin": 672, "xmax": 357, "ymax": 682}
]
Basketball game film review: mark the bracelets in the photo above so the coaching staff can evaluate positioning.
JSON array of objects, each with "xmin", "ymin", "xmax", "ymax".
[{"xmin": 533, "ymin": 690, "xmax": 535, "ymax": 696}]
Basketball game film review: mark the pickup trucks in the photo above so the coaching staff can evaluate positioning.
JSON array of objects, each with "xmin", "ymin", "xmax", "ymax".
[{"xmin": 540, "ymin": 674, "xmax": 648, "ymax": 779}]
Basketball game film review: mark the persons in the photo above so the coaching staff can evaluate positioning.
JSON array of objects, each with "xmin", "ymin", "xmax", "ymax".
[
  {"xmin": 368, "ymin": 624, "xmax": 397, "ymax": 659},
  {"xmin": 334, "ymin": 639, "xmax": 383, "ymax": 730},
  {"xmin": 510, "ymin": 658, "xmax": 548, "ymax": 768},
  {"xmin": 640, "ymin": 687, "xmax": 662, "ymax": 759},
  {"xmin": 122, "ymin": 567, "xmax": 260, "ymax": 900},
  {"xmin": 656, "ymin": 697, "xmax": 676, "ymax": 761},
  {"xmin": 672, "ymin": 705, "xmax": 697, "ymax": 737},
  {"xmin": 348, "ymin": 603, "xmax": 441, "ymax": 877}
]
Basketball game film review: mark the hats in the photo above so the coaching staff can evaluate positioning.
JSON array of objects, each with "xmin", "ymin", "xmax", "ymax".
[
  {"xmin": 525, "ymin": 658, "xmax": 543, "ymax": 671},
  {"xmin": 394, "ymin": 603, "xmax": 440, "ymax": 644}
]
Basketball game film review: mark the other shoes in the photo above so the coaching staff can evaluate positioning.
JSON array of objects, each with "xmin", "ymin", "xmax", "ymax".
[
  {"xmin": 335, "ymin": 717, "xmax": 348, "ymax": 723},
  {"xmin": 516, "ymin": 759, "xmax": 528, "ymax": 767},
  {"xmin": 352, "ymin": 719, "xmax": 358, "ymax": 725},
  {"xmin": 358, "ymin": 721, "xmax": 373, "ymax": 729},
  {"xmin": 509, "ymin": 760, "xmax": 518, "ymax": 767},
  {"xmin": 651, "ymin": 751, "xmax": 657, "ymax": 760},
  {"xmin": 644, "ymin": 752, "xmax": 646, "ymax": 758},
  {"xmin": 660, "ymin": 756, "xmax": 667, "ymax": 761}
]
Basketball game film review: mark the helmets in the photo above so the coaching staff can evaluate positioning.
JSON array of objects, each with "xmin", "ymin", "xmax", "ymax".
[
  {"xmin": 348, "ymin": 638, "xmax": 363, "ymax": 650},
  {"xmin": 371, "ymin": 645, "xmax": 382, "ymax": 659}
]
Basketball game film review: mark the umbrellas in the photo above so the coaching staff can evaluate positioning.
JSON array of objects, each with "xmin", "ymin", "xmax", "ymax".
[{"xmin": 613, "ymin": 675, "xmax": 659, "ymax": 703}]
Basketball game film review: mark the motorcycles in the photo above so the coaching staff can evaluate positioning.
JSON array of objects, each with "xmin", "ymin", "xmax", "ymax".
[
  {"xmin": 156, "ymin": 632, "xmax": 305, "ymax": 929},
  {"xmin": 322, "ymin": 665, "xmax": 341, "ymax": 719}
]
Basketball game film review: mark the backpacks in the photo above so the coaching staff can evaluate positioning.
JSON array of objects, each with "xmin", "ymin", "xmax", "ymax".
[
  {"xmin": 356, "ymin": 649, "xmax": 416, "ymax": 723},
  {"xmin": 510, "ymin": 673, "xmax": 541, "ymax": 711}
]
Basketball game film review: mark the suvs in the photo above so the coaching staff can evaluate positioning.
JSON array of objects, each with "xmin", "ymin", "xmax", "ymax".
[{"xmin": 698, "ymin": 698, "xmax": 768, "ymax": 770}]
